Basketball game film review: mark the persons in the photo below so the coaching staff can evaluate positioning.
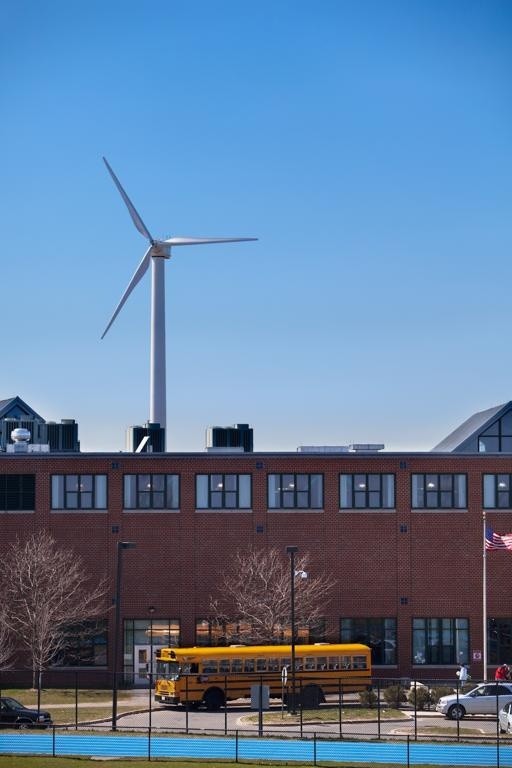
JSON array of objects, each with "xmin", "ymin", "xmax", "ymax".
[
  {"xmin": 458, "ymin": 663, "xmax": 468, "ymax": 686},
  {"xmin": 495, "ymin": 664, "xmax": 512, "ymax": 681}
]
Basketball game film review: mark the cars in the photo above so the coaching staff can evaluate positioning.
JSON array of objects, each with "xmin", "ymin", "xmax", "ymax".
[
  {"xmin": 498, "ymin": 700, "xmax": 512, "ymax": 735},
  {"xmin": 435, "ymin": 683, "xmax": 512, "ymax": 721}
]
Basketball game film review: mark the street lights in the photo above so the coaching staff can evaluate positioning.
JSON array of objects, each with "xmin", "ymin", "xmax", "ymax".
[
  {"xmin": 148, "ymin": 606, "xmax": 156, "ymax": 674},
  {"xmin": 290, "ymin": 567, "xmax": 308, "ymax": 641}
]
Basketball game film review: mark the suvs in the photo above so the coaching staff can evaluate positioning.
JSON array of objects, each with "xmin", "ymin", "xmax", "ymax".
[{"xmin": 1, "ymin": 697, "xmax": 52, "ymax": 730}]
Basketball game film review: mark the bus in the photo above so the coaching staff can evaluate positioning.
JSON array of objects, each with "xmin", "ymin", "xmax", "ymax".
[{"xmin": 154, "ymin": 643, "xmax": 373, "ymax": 710}]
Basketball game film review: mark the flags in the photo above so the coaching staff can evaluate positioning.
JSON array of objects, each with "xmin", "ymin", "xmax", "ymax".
[{"xmin": 485, "ymin": 527, "xmax": 512, "ymax": 550}]
